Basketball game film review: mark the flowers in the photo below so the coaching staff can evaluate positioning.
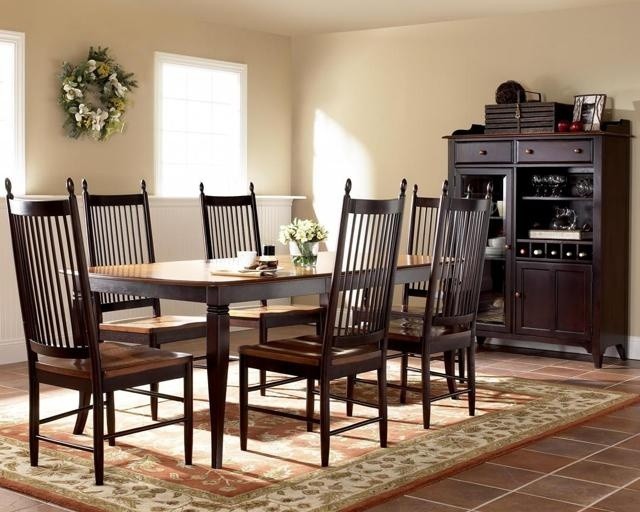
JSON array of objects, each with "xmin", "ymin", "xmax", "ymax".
[
  {"xmin": 55, "ymin": 46, "xmax": 140, "ymax": 145},
  {"xmin": 278, "ymin": 216, "xmax": 328, "ymax": 264}
]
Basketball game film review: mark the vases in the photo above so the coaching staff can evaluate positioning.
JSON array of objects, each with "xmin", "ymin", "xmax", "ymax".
[{"xmin": 288, "ymin": 241, "xmax": 319, "ymax": 265}]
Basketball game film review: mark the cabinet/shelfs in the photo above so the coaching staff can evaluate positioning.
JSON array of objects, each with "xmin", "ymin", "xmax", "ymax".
[{"xmin": 434, "ymin": 128, "xmax": 633, "ymax": 371}]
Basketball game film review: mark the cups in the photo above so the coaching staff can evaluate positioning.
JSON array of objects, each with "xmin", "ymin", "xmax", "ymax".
[
  {"xmin": 497, "ymin": 200, "xmax": 503, "ymax": 217},
  {"xmin": 236, "ymin": 250, "xmax": 257, "ymax": 268}
]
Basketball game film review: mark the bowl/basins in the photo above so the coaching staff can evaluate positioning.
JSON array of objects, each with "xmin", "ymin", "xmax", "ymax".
[
  {"xmin": 487, "ymin": 237, "xmax": 506, "ymax": 247},
  {"xmin": 484, "ymin": 247, "xmax": 508, "ymax": 258}
]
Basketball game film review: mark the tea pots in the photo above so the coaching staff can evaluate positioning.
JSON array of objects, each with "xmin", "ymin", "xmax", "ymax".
[
  {"xmin": 575, "ymin": 175, "xmax": 593, "ymax": 197},
  {"xmin": 558, "ymin": 119, "xmax": 584, "ymax": 133},
  {"xmin": 552, "ymin": 206, "xmax": 578, "ymax": 230}
]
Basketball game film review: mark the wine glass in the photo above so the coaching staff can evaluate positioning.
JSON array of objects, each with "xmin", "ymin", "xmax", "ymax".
[{"xmin": 531, "ymin": 175, "xmax": 569, "ymax": 198}]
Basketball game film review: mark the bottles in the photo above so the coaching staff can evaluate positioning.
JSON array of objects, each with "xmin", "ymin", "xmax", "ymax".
[
  {"xmin": 519, "ymin": 248, "xmax": 592, "ymax": 260},
  {"xmin": 259, "ymin": 245, "xmax": 279, "ymax": 268}
]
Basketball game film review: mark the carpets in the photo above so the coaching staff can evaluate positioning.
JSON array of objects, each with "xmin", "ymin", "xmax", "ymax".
[{"xmin": 2, "ymin": 340, "xmax": 639, "ymax": 510}]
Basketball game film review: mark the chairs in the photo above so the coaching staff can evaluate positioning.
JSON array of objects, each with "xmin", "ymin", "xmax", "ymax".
[
  {"xmin": 350, "ymin": 180, "xmax": 474, "ymax": 384},
  {"xmin": 242, "ymin": 179, "xmax": 407, "ymax": 465},
  {"xmin": 3, "ymin": 179, "xmax": 193, "ymax": 480},
  {"xmin": 195, "ymin": 181, "xmax": 321, "ymax": 395},
  {"xmin": 80, "ymin": 180, "xmax": 209, "ymax": 437},
  {"xmin": 347, "ymin": 179, "xmax": 495, "ymax": 433}
]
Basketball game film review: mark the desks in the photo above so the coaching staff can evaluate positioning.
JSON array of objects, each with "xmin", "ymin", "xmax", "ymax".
[{"xmin": 64, "ymin": 252, "xmax": 462, "ymax": 471}]
